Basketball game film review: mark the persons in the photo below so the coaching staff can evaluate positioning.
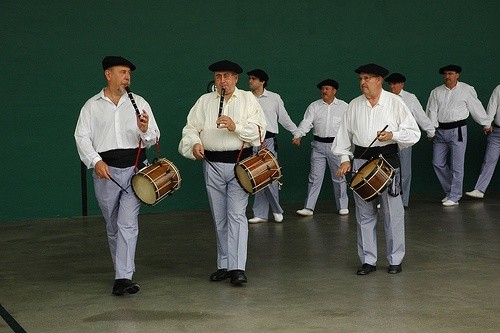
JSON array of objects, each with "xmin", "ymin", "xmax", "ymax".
[
  {"xmin": 331, "ymin": 64, "xmax": 421, "ymax": 275},
  {"xmin": 248, "ymin": 69, "xmax": 300, "ymax": 223},
  {"xmin": 74, "ymin": 57, "xmax": 160, "ymax": 295},
  {"xmin": 426, "ymin": 65, "xmax": 490, "ymax": 206},
  {"xmin": 384, "ymin": 73, "xmax": 435, "ymax": 209},
  {"xmin": 466, "ymin": 84, "xmax": 500, "ymax": 198},
  {"xmin": 178, "ymin": 60, "xmax": 267, "ymax": 282},
  {"xmin": 292, "ymin": 80, "xmax": 349, "ymax": 216}
]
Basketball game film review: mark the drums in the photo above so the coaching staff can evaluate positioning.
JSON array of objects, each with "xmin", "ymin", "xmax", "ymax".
[
  {"xmin": 131, "ymin": 158, "xmax": 182, "ymax": 206},
  {"xmin": 234, "ymin": 148, "xmax": 284, "ymax": 194},
  {"xmin": 349, "ymin": 154, "xmax": 396, "ymax": 204}
]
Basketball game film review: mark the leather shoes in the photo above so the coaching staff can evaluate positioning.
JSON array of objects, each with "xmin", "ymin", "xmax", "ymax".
[
  {"xmin": 111, "ymin": 279, "xmax": 141, "ymax": 296},
  {"xmin": 230, "ymin": 270, "xmax": 248, "ymax": 284},
  {"xmin": 210, "ymin": 269, "xmax": 230, "ymax": 281},
  {"xmin": 356, "ymin": 263, "xmax": 376, "ymax": 275},
  {"xmin": 388, "ymin": 263, "xmax": 402, "ymax": 274}
]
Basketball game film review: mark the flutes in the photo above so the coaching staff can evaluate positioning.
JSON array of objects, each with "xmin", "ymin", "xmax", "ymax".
[
  {"xmin": 124, "ymin": 85, "xmax": 141, "ymax": 118},
  {"xmin": 216, "ymin": 87, "xmax": 225, "ymax": 129}
]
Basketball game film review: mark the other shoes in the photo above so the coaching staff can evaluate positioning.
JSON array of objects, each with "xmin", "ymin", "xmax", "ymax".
[
  {"xmin": 465, "ymin": 189, "xmax": 484, "ymax": 198},
  {"xmin": 442, "ymin": 199, "xmax": 459, "ymax": 206},
  {"xmin": 248, "ymin": 217, "xmax": 267, "ymax": 223},
  {"xmin": 377, "ymin": 203, "xmax": 380, "ymax": 209},
  {"xmin": 441, "ymin": 197, "xmax": 449, "ymax": 202},
  {"xmin": 273, "ymin": 212, "xmax": 283, "ymax": 222},
  {"xmin": 296, "ymin": 208, "xmax": 313, "ymax": 215},
  {"xmin": 339, "ymin": 208, "xmax": 349, "ymax": 215}
]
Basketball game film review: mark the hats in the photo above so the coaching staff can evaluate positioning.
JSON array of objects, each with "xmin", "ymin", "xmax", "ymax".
[
  {"xmin": 208, "ymin": 60, "xmax": 243, "ymax": 74},
  {"xmin": 384, "ymin": 73, "xmax": 406, "ymax": 83},
  {"xmin": 317, "ymin": 79, "xmax": 338, "ymax": 90},
  {"xmin": 247, "ymin": 69, "xmax": 269, "ymax": 82},
  {"xmin": 102, "ymin": 56, "xmax": 136, "ymax": 71},
  {"xmin": 438, "ymin": 64, "xmax": 462, "ymax": 73},
  {"xmin": 354, "ymin": 64, "xmax": 389, "ymax": 77}
]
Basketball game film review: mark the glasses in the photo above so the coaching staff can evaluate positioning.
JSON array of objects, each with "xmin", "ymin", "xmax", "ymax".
[{"xmin": 358, "ymin": 76, "xmax": 375, "ymax": 81}]
